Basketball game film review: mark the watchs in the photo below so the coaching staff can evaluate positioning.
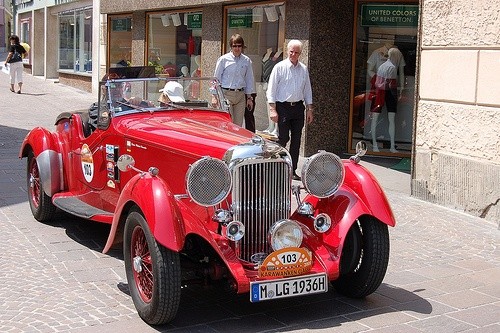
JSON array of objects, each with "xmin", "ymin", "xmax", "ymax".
[{"xmin": 247, "ymin": 97, "xmax": 253, "ymax": 100}]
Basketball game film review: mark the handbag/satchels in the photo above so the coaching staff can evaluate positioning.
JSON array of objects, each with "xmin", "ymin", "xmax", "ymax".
[{"xmin": 8, "ymin": 45, "xmax": 19, "ymax": 63}]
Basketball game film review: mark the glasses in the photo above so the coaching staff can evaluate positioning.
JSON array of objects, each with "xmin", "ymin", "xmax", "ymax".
[
  {"xmin": 232, "ymin": 45, "xmax": 242, "ymax": 47},
  {"xmin": 110, "ymin": 83, "xmax": 125, "ymax": 89}
]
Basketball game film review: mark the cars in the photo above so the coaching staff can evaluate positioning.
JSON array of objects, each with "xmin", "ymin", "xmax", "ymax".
[
  {"xmin": 353, "ymin": 88, "xmax": 412, "ymax": 139},
  {"xmin": 18, "ymin": 76, "xmax": 395, "ymax": 328}
]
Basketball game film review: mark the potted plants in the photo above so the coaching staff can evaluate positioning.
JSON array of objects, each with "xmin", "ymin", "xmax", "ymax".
[{"xmin": 148, "ymin": 56, "xmax": 169, "ymax": 93}]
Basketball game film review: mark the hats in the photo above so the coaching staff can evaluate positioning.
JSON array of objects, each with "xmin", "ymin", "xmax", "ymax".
[{"xmin": 163, "ymin": 81, "xmax": 186, "ymax": 103}]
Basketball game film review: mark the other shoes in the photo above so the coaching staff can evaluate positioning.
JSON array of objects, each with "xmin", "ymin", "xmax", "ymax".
[
  {"xmin": 10, "ymin": 89, "xmax": 14, "ymax": 92},
  {"xmin": 17, "ymin": 90, "xmax": 21, "ymax": 94},
  {"xmin": 292, "ymin": 173, "xmax": 301, "ymax": 180}
]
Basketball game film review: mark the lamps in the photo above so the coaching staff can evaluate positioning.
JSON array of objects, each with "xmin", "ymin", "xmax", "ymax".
[
  {"xmin": 252, "ymin": 5, "xmax": 263, "ymax": 23},
  {"xmin": 171, "ymin": 13, "xmax": 181, "ymax": 26},
  {"xmin": 184, "ymin": 12, "xmax": 187, "ymax": 26},
  {"xmin": 161, "ymin": 15, "xmax": 170, "ymax": 27},
  {"xmin": 279, "ymin": 4, "xmax": 286, "ymax": 21},
  {"xmin": 264, "ymin": 5, "xmax": 279, "ymax": 22}
]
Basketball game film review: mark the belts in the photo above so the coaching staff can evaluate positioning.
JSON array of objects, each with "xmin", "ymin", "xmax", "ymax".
[
  {"xmin": 276, "ymin": 101, "xmax": 303, "ymax": 106},
  {"xmin": 222, "ymin": 88, "xmax": 243, "ymax": 91}
]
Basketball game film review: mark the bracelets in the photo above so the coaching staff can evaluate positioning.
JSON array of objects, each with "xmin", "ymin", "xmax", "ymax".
[{"xmin": 307, "ymin": 108, "xmax": 313, "ymax": 111}]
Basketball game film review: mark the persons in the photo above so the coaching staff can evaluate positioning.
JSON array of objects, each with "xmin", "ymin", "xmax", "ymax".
[
  {"xmin": 266, "ymin": 39, "xmax": 314, "ymax": 180},
  {"xmin": 212, "ymin": 34, "xmax": 254, "ymax": 127},
  {"xmin": 272, "ymin": 47, "xmax": 283, "ymax": 66},
  {"xmin": 261, "ymin": 46, "xmax": 276, "ymax": 82},
  {"xmin": 241, "ymin": 46, "xmax": 255, "ymax": 133},
  {"xmin": 88, "ymin": 73, "xmax": 153, "ymax": 133},
  {"xmin": 366, "ymin": 44, "xmax": 405, "ymax": 154},
  {"xmin": 180, "ymin": 66, "xmax": 192, "ymax": 99},
  {"xmin": 192, "ymin": 55, "xmax": 201, "ymax": 99},
  {"xmin": 161, "ymin": 80, "xmax": 185, "ymax": 104},
  {"xmin": 375, "ymin": 48, "xmax": 402, "ymax": 89},
  {"xmin": 4, "ymin": 35, "xmax": 28, "ymax": 94}
]
